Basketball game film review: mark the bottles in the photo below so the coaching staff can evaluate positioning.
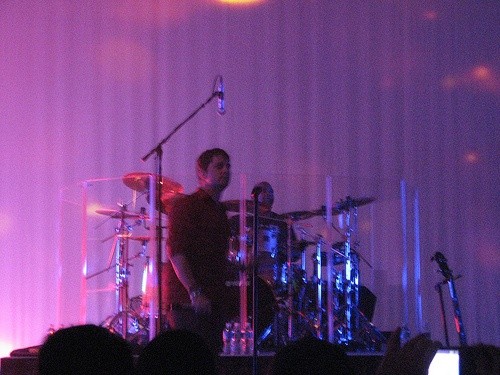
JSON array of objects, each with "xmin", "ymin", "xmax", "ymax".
[
  {"xmin": 399, "ymin": 320, "xmax": 411, "ymax": 348},
  {"xmin": 223, "ymin": 322, "xmax": 255, "ymax": 357}
]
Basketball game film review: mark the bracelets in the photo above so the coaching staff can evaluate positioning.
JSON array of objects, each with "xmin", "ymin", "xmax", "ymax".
[{"xmin": 189, "ymin": 289, "xmax": 202, "ymax": 299}]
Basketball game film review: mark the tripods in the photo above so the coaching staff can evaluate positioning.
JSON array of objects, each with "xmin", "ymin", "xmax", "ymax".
[
  {"xmin": 257, "ymin": 218, "xmax": 391, "ymax": 353},
  {"xmin": 98, "ymin": 221, "xmax": 151, "ymax": 343}
]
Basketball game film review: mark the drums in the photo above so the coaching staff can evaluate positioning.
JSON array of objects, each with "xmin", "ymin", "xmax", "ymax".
[
  {"xmin": 221, "ymin": 270, "xmax": 279, "ymax": 346},
  {"xmin": 139, "ymin": 261, "xmax": 173, "ymax": 317},
  {"xmin": 235, "ymin": 218, "xmax": 280, "ymax": 267}
]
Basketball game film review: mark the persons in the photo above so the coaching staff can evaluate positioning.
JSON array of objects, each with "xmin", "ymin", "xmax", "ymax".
[
  {"xmin": 230, "ymin": 181, "xmax": 306, "ymax": 262},
  {"xmin": 162, "ymin": 148, "xmax": 232, "ymax": 352},
  {"xmin": 40, "ymin": 323, "xmax": 443, "ymax": 375}
]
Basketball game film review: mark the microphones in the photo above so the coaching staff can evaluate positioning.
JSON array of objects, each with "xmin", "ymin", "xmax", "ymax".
[
  {"xmin": 169, "ymin": 302, "xmax": 195, "ymax": 311},
  {"xmin": 216, "ymin": 76, "xmax": 225, "ymax": 115},
  {"xmin": 435, "ymin": 252, "xmax": 453, "ymax": 279}
]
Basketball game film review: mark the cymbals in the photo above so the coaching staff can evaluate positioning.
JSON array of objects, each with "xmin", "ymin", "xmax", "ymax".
[
  {"xmin": 146, "ymin": 192, "xmax": 189, "ymax": 215},
  {"xmin": 222, "ymin": 199, "xmax": 254, "ymax": 212},
  {"xmin": 115, "ymin": 234, "xmax": 167, "ymax": 244},
  {"xmin": 96, "ymin": 208, "xmax": 150, "ymax": 220},
  {"xmin": 282, "ymin": 196, "xmax": 379, "ymax": 220},
  {"xmin": 124, "ymin": 172, "xmax": 184, "ymax": 195}
]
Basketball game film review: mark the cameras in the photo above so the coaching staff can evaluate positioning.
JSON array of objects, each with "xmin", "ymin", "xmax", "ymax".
[{"xmin": 428, "ymin": 349, "xmax": 461, "ymax": 375}]
